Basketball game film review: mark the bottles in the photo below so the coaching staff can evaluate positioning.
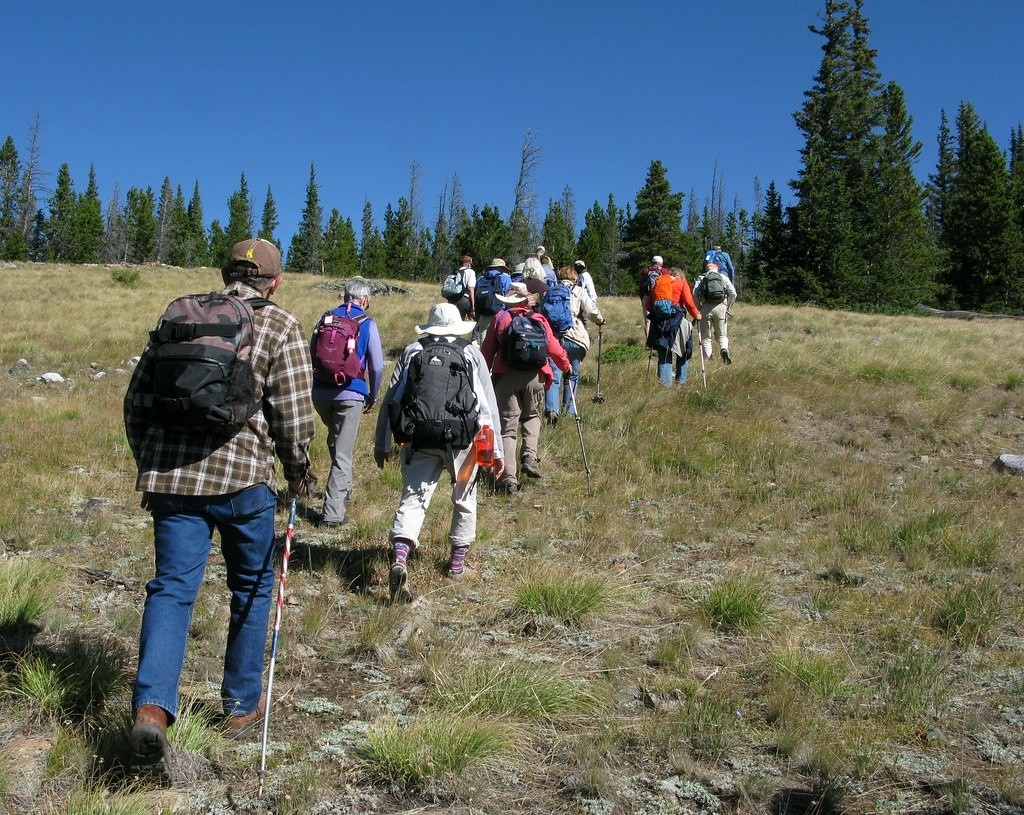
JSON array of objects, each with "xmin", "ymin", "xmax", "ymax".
[{"xmin": 475, "ymin": 425, "xmax": 494, "ymax": 467}]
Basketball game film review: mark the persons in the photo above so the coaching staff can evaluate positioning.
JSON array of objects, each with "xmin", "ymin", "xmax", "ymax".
[
  {"xmin": 510, "ymin": 262, "xmax": 525, "ymax": 281},
  {"xmin": 518, "ymin": 257, "xmax": 551, "ymax": 298},
  {"xmin": 371, "ymin": 303, "xmax": 506, "ymax": 607},
  {"xmin": 541, "ymin": 257, "xmax": 558, "ymax": 287},
  {"xmin": 481, "ymin": 281, "xmax": 573, "ymax": 495},
  {"xmin": 574, "ymin": 260, "xmax": 598, "ymax": 325},
  {"xmin": 646, "ymin": 267, "xmax": 703, "ymax": 388},
  {"xmin": 448, "ymin": 255, "xmax": 475, "ymax": 322},
  {"xmin": 692, "ymin": 263, "xmax": 738, "ymax": 364},
  {"xmin": 474, "ymin": 258, "xmax": 512, "ymax": 350},
  {"xmin": 702, "ymin": 245, "xmax": 735, "ymax": 295},
  {"xmin": 539, "ymin": 267, "xmax": 606, "ymax": 428},
  {"xmin": 123, "ymin": 238, "xmax": 318, "ymax": 791},
  {"xmin": 638, "ymin": 255, "xmax": 669, "ymax": 339},
  {"xmin": 309, "ymin": 278, "xmax": 384, "ymax": 529},
  {"xmin": 536, "ymin": 245, "xmax": 555, "ymax": 269}
]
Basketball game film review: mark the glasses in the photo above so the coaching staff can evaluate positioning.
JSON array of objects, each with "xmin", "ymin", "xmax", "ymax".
[{"xmin": 365, "ymin": 299, "xmax": 369, "ymax": 310}]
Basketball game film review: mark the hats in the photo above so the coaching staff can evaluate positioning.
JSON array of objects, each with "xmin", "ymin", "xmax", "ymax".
[
  {"xmin": 494, "ymin": 282, "xmax": 539, "ymax": 304},
  {"xmin": 487, "ymin": 258, "xmax": 510, "ymax": 272},
  {"xmin": 574, "ymin": 260, "xmax": 586, "ymax": 267},
  {"xmin": 536, "ymin": 246, "xmax": 545, "ymax": 251},
  {"xmin": 511, "ymin": 263, "xmax": 526, "ymax": 274},
  {"xmin": 228, "ymin": 238, "xmax": 282, "ymax": 276},
  {"xmin": 652, "ymin": 256, "xmax": 664, "ymax": 262},
  {"xmin": 460, "ymin": 255, "xmax": 475, "ymax": 268}
]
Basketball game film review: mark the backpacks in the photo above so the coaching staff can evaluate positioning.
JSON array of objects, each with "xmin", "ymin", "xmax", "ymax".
[
  {"xmin": 148, "ymin": 291, "xmax": 281, "ymax": 427},
  {"xmin": 548, "ymin": 278, "xmax": 555, "ymax": 286},
  {"xmin": 542, "ymin": 285, "xmax": 574, "ymax": 331},
  {"xmin": 702, "ymin": 272, "xmax": 725, "ymax": 303},
  {"xmin": 712, "ymin": 251, "xmax": 728, "ymax": 272},
  {"xmin": 311, "ymin": 310, "xmax": 372, "ymax": 385},
  {"xmin": 389, "ymin": 337, "xmax": 481, "ymax": 450},
  {"xmin": 503, "ymin": 308, "xmax": 548, "ymax": 370},
  {"xmin": 578, "ymin": 274, "xmax": 584, "ymax": 287},
  {"xmin": 474, "ymin": 273, "xmax": 504, "ymax": 316},
  {"xmin": 651, "ymin": 274, "xmax": 675, "ymax": 314},
  {"xmin": 441, "ymin": 268, "xmax": 473, "ymax": 301},
  {"xmin": 645, "ymin": 266, "xmax": 663, "ymax": 293}
]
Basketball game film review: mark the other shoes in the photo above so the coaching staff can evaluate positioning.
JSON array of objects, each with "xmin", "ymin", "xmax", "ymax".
[
  {"xmin": 721, "ymin": 349, "xmax": 731, "ymax": 364},
  {"xmin": 222, "ymin": 694, "xmax": 267, "ymax": 738},
  {"xmin": 520, "ymin": 455, "xmax": 542, "ymax": 478},
  {"xmin": 504, "ymin": 484, "xmax": 518, "ymax": 494},
  {"xmin": 546, "ymin": 410, "xmax": 558, "ymax": 428},
  {"xmin": 344, "ymin": 489, "xmax": 352, "ymax": 499},
  {"xmin": 388, "ymin": 559, "xmax": 411, "ymax": 605},
  {"xmin": 126, "ymin": 704, "xmax": 173, "ymax": 791},
  {"xmin": 324, "ymin": 515, "xmax": 349, "ymax": 526},
  {"xmin": 446, "ymin": 565, "xmax": 464, "ymax": 579}
]
